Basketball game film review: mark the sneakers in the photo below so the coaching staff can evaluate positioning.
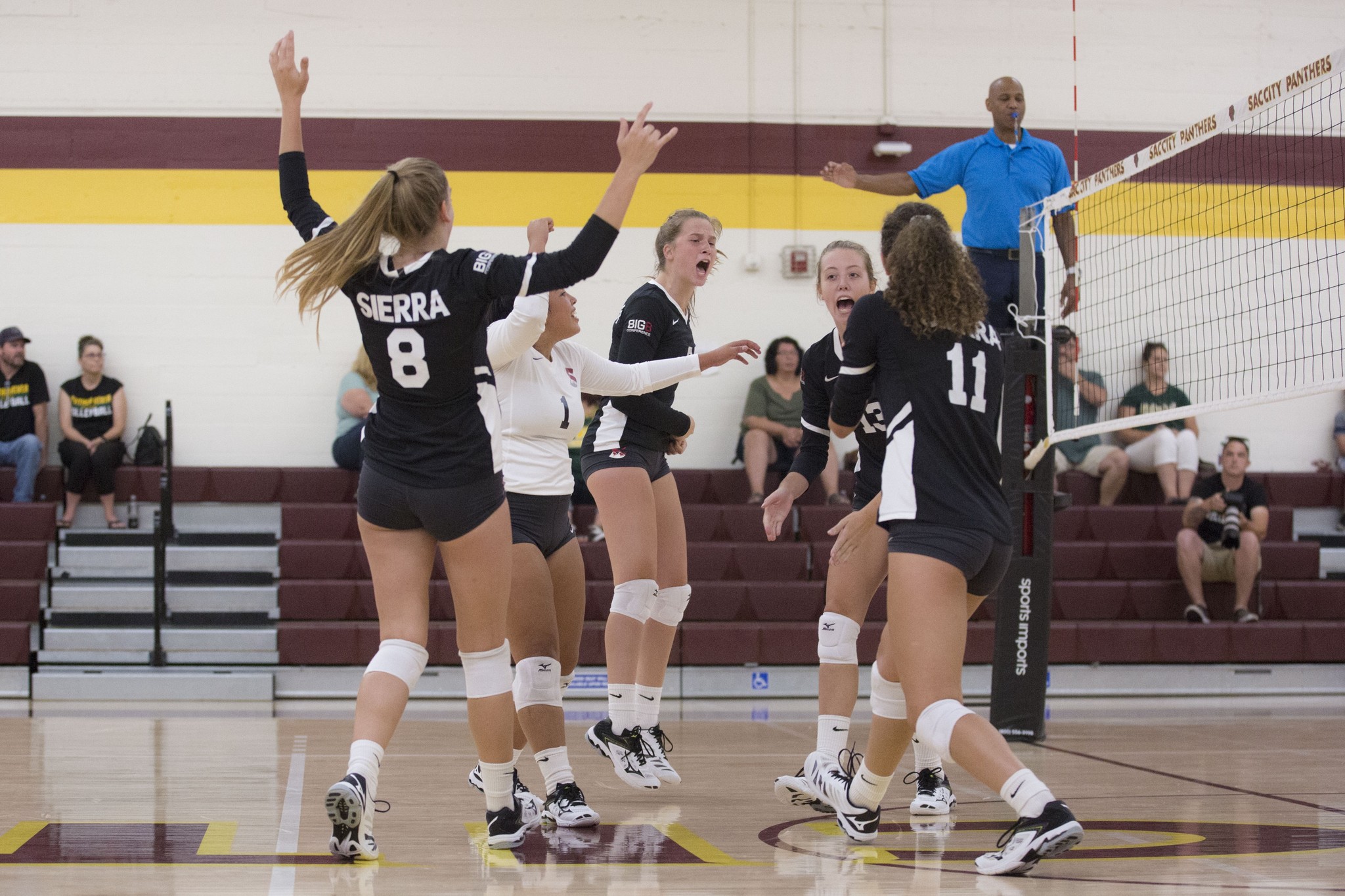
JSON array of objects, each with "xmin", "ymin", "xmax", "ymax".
[
  {"xmin": 638, "ymin": 721, "xmax": 682, "ymax": 785},
  {"xmin": 542, "ymin": 781, "xmax": 600, "ymax": 827},
  {"xmin": 903, "ymin": 767, "xmax": 957, "ymax": 816},
  {"xmin": 804, "ymin": 742, "xmax": 880, "ymax": 842},
  {"xmin": 974, "ymin": 801, "xmax": 1084, "ymax": 875},
  {"xmin": 542, "ymin": 826, "xmax": 600, "ymax": 854},
  {"xmin": 325, "ymin": 773, "xmax": 390, "ymax": 860},
  {"xmin": 910, "ymin": 813, "xmax": 951, "ymax": 836},
  {"xmin": 774, "ymin": 767, "xmax": 838, "ymax": 814},
  {"xmin": 584, "ymin": 716, "xmax": 660, "ymax": 791},
  {"xmin": 615, "ymin": 804, "xmax": 679, "ymax": 855},
  {"xmin": 467, "ymin": 763, "xmax": 545, "ymax": 812},
  {"xmin": 486, "ymin": 794, "xmax": 542, "ymax": 850}
]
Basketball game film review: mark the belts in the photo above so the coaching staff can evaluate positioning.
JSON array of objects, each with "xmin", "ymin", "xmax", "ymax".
[{"xmin": 967, "ymin": 245, "xmax": 1041, "ymax": 260}]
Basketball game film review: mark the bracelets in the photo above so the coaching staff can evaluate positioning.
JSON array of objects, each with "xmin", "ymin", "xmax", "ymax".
[{"xmin": 99, "ymin": 433, "xmax": 109, "ymax": 444}]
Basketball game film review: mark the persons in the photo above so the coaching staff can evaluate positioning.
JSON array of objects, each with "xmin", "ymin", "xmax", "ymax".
[
  {"xmin": 1334, "ymin": 387, "xmax": 1345, "ymax": 532},
  {"xmin": 268, "ymin": 27, "xmax": 678, "ymax": 857},
  {"xmin": 1175, "ymin": 438, "xmax": 1269, "ymax": 623},
  {"xmin": 1115, "ymin": 342, "xmax": 1199, "ymax": 506},
  {"xmin": 757, "ymin": 239, "xmax": 958, "ymax": 816},
  {"xmin": 564, "ymin": 391, "xmax": 607, "ymax": 545},
  {"xmin": 1050, "ymin": 325, "xmax": 1129, "ymax": 508},
  {"xmin": 55, "ymin": 336, "xmax": 129, "ymax": 530},
  {"xmin": 330, "ymin": 346, "xmax": 382, "ymax": 470},
  {"xmin": 732, "ymin": 335, "xmax": 852, "ymax": 506},
  {"xmin": 578, "ymin": 211, "xmax": 728, "ymax": 794},
  {"xmin": 804, "ymin": 201, "xmax": 1085, "ymax": 877},
  {"xmin": 0, "ymin": 327, "xmax": 52, "ymax": 504},
  {"xmin": 820, "ymin": 77, "xmax": 1082, "ymax": 335},
  {"xmin": 469, "ymin": 219, "xmax": 764, "ymax": 827}
]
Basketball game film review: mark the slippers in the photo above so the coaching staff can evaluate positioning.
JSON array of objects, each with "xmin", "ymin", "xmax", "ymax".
[
  {"xmin": 109, "ymin": 519, "xmax": 126, "ymax": 529},
  {"xmin": 56, "ymin": 520, "xmax": 72, "ymax": 528}
]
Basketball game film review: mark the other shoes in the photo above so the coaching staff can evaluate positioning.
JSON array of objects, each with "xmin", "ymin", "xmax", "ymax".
[
  {"xmin": 1185, "ymin": 605, "xmax": 1212, "ymax": 625},
  {"xmin": 1234, "ymin": 608, "xmax": 1259, "ymax": 624},
  {"xmin": 587, "ymin": 524, "xmax": 607, "ymax": 543}
]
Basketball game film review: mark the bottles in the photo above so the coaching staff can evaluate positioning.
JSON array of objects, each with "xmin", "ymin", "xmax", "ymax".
[{"xmin": 128, "ymin": 495, "xmax": 138, "ymax": 529}]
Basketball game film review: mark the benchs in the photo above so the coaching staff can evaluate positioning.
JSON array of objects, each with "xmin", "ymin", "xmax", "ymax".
[{"xmin": 0, "ymin": 461, "xmax": 1345, "ymax": 691}]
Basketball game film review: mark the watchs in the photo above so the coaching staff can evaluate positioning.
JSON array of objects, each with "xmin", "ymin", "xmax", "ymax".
[{"xmin": 1066, "ymin": 267, "xmax": 1082, "ymax": 279}]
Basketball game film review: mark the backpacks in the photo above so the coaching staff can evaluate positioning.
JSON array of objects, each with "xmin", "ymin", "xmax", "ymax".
[{"xmin": 126, "ymin": 414, "xmax": 163, "ymax": 467}]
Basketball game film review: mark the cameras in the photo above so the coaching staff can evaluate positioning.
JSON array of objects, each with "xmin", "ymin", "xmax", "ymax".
[{"xmin": 1217, "ymin": 491, "xmax": 1245, "ymax": 548}]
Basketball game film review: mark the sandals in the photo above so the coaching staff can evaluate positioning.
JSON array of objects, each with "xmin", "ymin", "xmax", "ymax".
[
  {"xmin": 746, "ymin": 490, "xmax": 766, "ymax": 505},
  {"xmin": 826, "ymin": 490, "xmax": 852, "ymax": 504}
]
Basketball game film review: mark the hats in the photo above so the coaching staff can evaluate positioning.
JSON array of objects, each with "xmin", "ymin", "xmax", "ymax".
[{"xmin": 0, "ymin": 327, "xmax": 31, "ymax": 347}]
